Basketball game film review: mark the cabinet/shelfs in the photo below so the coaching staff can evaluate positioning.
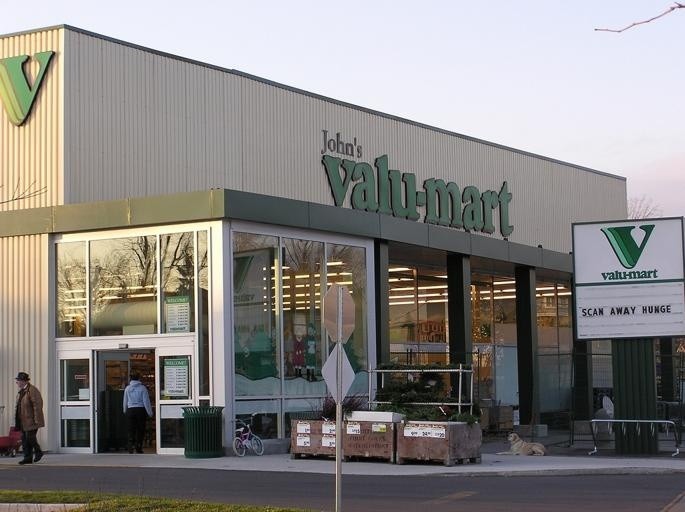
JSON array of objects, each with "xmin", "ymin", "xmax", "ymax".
[{"xmin": 368, "ymin": 362, "xmax": 476, "ymax": 415}]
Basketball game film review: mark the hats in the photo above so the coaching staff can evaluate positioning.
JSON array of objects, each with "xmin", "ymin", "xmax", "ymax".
[{"xmin": 14, "ymin": 372, "xmax": 31, "ymax": 381}]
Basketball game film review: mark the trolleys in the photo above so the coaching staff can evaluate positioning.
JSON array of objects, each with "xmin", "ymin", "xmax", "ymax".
[{"xmin": 0, "ymin": 426, "xmax": 22, "ymax": 457}]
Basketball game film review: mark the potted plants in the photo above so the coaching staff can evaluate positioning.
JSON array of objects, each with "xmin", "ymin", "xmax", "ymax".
[{"xmin": 290, "ymin": 371, "xmax": 484, "ymax": 465}]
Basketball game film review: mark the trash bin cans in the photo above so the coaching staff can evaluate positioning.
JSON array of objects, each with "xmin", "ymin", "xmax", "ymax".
[{"xmin": 181, "ymin": 406, "xmax": 226, "ymax": 458}]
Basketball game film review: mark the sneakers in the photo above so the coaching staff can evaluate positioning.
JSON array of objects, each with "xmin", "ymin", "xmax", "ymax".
[
  {"xmin": 34, "ymin": 453, "xmax": 43, "ymax": 462},
  {"xmin": 129, "ymin": 449, "xmax": 145, "ymax": 454},
  {"xmin": 18, "ymin": 458, "xmax": 33, "ymax": 465}
]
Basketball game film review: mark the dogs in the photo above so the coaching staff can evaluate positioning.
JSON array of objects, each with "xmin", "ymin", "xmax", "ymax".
[{"xmin": 496, "ymin": 433, "xmax": 550, "ymax": 456}]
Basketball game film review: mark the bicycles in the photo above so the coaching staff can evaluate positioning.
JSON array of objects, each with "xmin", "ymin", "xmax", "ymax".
[{"xmin": 232, "ymin": 412, "xmax": 264, "ymax": 457}]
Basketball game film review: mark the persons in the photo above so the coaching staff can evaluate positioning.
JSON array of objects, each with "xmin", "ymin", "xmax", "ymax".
[
  {"xmin": 13, "ymin": 371, "xmax": 45, "ymax": 464},
  {"xmin": 292, "ymin": 329, "xmax": 304, "ymax": 378},
  {"xmin": 122, "ymin": 370, "xmax": 154, "ymax": 454},
  {"xmin": 305, "ymin": 323, "xmax": 318, "ymax": 382}
]
